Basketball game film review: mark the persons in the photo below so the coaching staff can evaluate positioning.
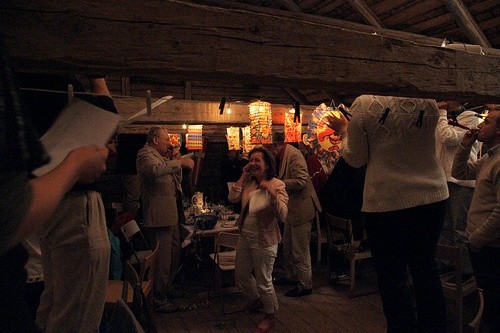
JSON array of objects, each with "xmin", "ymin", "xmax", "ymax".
[
  {"xmin": 435, "ymin": 98, "xmax": 500, "ymax": 333},
  {"xmin": 0, "ymin": 67, "xmax": 121, "ymax": 333},
  {"xmin": 269, "ymin": 130, "xmax": 323, "ymax": 297},
  {"xmin": 324, "ymin": 157, "xmax": 368, "ymax": 280},
  {"xmin": 136, "ymin": 125, "xmax": 194, "ymax": 313},
  {"xmin": 229, "ymin": 145, "xmax": 289, "ymax": 332},
  {"xmin": 324, "ymin": 95, "xmax": 450, "ymax": 333}
]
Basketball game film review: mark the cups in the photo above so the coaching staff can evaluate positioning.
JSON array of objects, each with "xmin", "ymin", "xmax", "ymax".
[
  {"xmin": 217, "ymin": 201, "xmax": 234, "ymax": 222},
  {"xmin": 191, "ymin": 192, "xmax": 204, "ymax": 209}
]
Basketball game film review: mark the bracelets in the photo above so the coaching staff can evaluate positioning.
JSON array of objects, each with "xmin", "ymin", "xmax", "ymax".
[{"xmin": 237, "ymin": 181, "xmax": 246, "ymax": 185}]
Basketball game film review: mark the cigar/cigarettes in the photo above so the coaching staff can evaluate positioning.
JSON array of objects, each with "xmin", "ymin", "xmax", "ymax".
[{"xmin": 471, "ymin": 129, "xmax": 479, "ymax": 131}]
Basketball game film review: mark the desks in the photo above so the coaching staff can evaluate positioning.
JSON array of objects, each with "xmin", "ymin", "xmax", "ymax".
[{"xmin": 179, "ymin": 218, "xmax": 240, "ymax": 273}]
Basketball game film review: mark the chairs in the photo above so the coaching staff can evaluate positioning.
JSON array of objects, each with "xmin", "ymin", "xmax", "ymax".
[
  {"xmin": 207, "ymin": 232, "xmax": 240, "ymax": 313},
  {"xmin": 312, "ymin": 211, "xmax": 372, "ymax": 289},
  {"xmin": 106, "ymin": 219, "xmax": 165, "ymax": 333},
  {"xmin": 437, "ymin": 242, "xmax": 484, "ymax": 333}
]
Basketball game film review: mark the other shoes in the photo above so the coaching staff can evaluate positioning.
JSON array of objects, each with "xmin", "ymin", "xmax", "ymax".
[
  {"xmin": 155, "ymin": 304, "xmax": 176, "ymax": 312},
  {"xmin": 256, "ymin": 320, "xmax": 276, "ymax": 333}
]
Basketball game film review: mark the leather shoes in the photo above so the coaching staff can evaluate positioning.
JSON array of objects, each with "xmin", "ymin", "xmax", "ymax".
[{"xmin": 285, "ymin": 287, "xmax": 312, "ymax": 297}]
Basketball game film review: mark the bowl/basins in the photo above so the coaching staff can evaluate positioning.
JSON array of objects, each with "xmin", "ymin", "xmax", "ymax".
[{"xmin": 194, "ymin": 215, "xmax": 217, "ymax": 230}]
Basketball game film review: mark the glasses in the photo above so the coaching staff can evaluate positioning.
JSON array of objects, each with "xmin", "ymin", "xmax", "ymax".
[{"xmin": 156, "ymin": 136, "xmax": 169, "ymax": 142}]
